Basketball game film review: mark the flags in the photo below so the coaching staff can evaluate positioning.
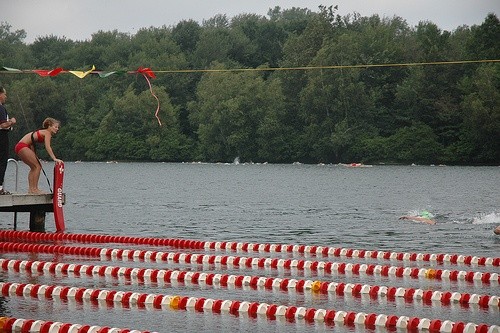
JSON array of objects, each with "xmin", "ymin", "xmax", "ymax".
[{"xmin": 31, "ymin": 65, "xmax": 156, "ymax": 79}]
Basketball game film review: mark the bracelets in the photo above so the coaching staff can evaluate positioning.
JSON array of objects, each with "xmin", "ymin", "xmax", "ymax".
[{"xmin": 9, "ymin": 119, "xmax": 13, "ymax": 125}]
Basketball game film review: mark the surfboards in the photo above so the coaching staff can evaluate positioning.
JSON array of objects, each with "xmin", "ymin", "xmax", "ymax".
[{"xmin": 53, "ymin": 162, "xmax": 64, "ymax": 232}]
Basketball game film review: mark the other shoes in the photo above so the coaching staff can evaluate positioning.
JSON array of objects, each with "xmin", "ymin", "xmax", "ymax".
[{"xmin": 0, "ymin": 186, "xmax": 13, "ymax": 195}]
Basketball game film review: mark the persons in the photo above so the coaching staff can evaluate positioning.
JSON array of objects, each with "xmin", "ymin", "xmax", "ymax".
[
  {"xmin": 494, "ymin": 226, "xmax": 500, "ymax": 235},
  {"xmin": 399, "ymin": 211, "xmax": 433, "ymax": 225},
  {"xmin": 0, "ymin": 86, "xmax": 16, "ymax": 195},
  {"xmin": 15, "ymin": 117, "xmax": 63, "ymax": 194}
]
